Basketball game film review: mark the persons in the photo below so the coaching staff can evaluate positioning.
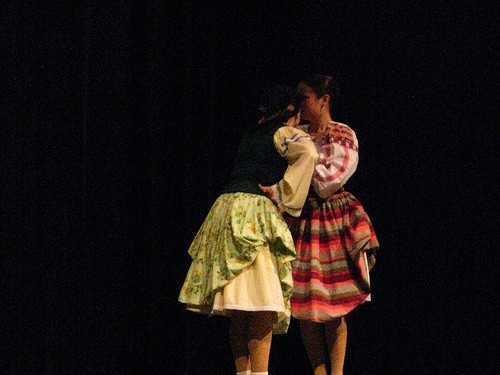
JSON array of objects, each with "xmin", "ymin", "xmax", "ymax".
[
  {"xmin": 176, "ymin": 85, "xmax": 320, "ymax": 374},
  {"xmin": 283, "ymin": 75, "xmax": 380, "ymax": 375}
]
